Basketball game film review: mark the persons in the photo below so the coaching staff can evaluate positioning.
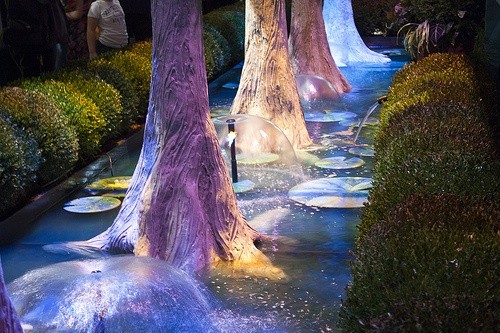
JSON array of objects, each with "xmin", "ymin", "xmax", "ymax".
[
  {"xmin": 86, "ymin": 0, "xmax": 129, "ymax": 61},
  {"xmin": 64, "ymin": 0, "xmax": 90, "ymax": 63}
]
